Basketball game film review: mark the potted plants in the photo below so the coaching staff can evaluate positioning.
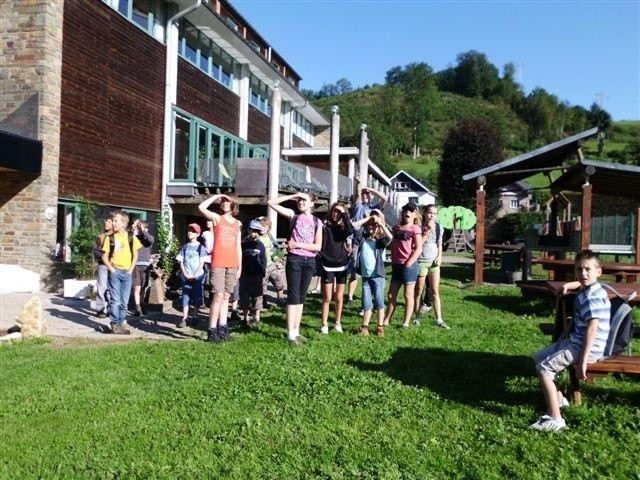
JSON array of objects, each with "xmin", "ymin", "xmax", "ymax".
[{"xmin": 63, "ymin": 195, "xmax": 98, "ymax": 298}]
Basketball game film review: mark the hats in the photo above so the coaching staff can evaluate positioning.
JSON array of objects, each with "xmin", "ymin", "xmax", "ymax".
[
  {"xmin": 402, "ymin": 203, "xmax": 419, "ymax": 213},
  {"xmin": 248, "ymin": 220, "xmax": 262, "ymax": 231},
  {"xmin": 188, "ymin": 223, "xmax": 200, "ymax": 233}
]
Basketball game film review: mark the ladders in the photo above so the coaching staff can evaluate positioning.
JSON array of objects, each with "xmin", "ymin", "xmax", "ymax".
[{"xmin": 451, "ymin": 208, "xmax": 467, "ymax": 253}]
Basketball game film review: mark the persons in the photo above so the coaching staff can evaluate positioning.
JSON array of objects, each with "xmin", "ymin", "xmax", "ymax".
[
  {"xmin": 266, "ymin": 189, "xmax": 323, "ymax": 347},
  {"xmin": 315, "ymin": 181, "xmax": 451, "ymax": 338},
  {"xmin": 531, "ymin": 249, "xmax": 613, "ymax": 431},
  {"xmin": 175, "ymin": 192, "xmax": 284, "ymax": 344},
  {"xmin": 94, "ymin": 207, "xmax": 167, "ymax": 335}
]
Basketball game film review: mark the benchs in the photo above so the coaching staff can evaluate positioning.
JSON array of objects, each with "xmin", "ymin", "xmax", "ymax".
[
  {"xmin": 515, "ymin": 277, "xmax": 640, "ymax": 406},
  {"xmin": 474, "ymin": 255, "xmax": 500, "ymax": 265}
]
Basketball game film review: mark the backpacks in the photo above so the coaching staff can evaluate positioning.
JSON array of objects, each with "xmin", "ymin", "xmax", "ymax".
[{"xmin": 600, "ymin": 282, "xmax": 638, "ymax": 356}]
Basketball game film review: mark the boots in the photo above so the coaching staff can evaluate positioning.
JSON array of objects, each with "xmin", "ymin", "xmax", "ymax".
[
  {"xmin": 178, "ymin": 310, "xmax": 261, "ymax": 342},
  {"xmin": 96, "ymin": 309, "xmax": 147, "ymax": 334}
]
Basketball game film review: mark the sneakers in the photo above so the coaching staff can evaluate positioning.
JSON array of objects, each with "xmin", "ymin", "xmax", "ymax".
[
  {"xmin": 321, "ymin": 325, "xmax": 328, "ymax": 334},
  {"xmin": 334, "ymin": 325, "xmax": 343, "ymax": 333},
  {"xmin": 359, "ymin": 303, "xmax": 451, "ymax": 337},
  {"xmin": 559, "ymin": 397, "xmax": 570, "ymax": 408},
  {"xmin": 344, "ymin": 298, "xmax": 353, "ymax": 308},
  {"xmin": 288, "ymin": 336, "xmax": 309, "ymax": 345},
  {"xmin": 530, "ymin": 415, "xmax": 567, "ymax": 434},
  {"xmin": 278, "ymin": 297, "xmax": 288, "ymax": 304}
]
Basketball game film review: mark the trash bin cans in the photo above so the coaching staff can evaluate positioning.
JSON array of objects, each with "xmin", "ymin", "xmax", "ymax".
[{"xmin": 500, "ymin": 251, "xmax": 521, "ymax": 285}]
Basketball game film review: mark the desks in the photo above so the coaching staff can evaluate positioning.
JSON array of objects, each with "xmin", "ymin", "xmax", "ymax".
[
  {"xmin": 484, "ymin": 244, "xmax": 520, "ymax": 262},
  {"xmin": 540, "ymin": 260, "xmax": 639, "ymax": 283}
]
